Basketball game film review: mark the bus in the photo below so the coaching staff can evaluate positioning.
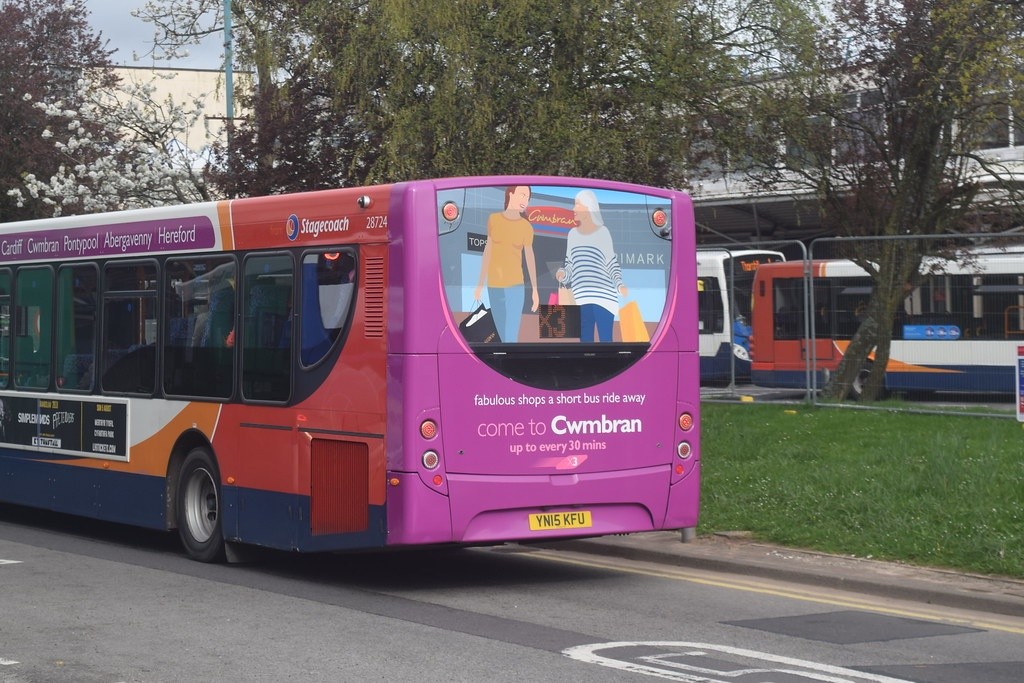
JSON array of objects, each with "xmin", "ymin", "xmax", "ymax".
[
  {"xmin": 0, "ymin": 175, "xmax": 702, "ymax": 566},
  {"xmin": 693, "ymin": 249, "xmax": 786, "ymax": 383},
  {"xmin": 748, "ymin": 245, "xmax": 1023, "ymax": 395}
]
